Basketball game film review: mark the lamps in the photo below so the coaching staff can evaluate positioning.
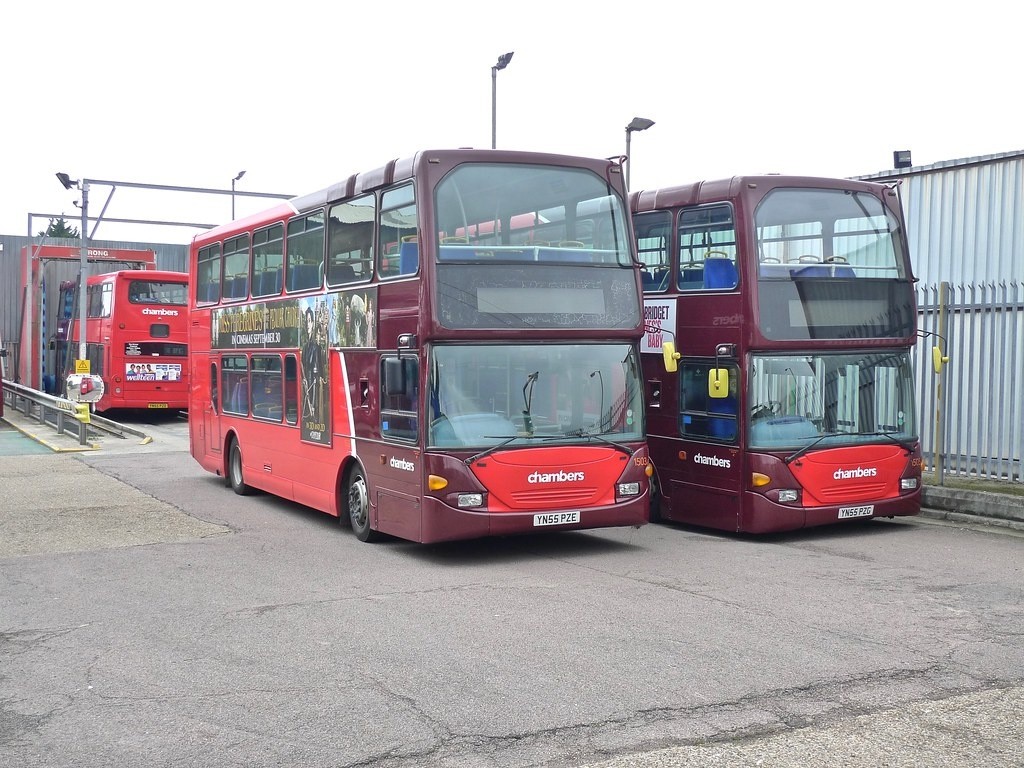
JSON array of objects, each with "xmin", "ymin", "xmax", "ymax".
[{"xmin": 893, "ymin": 151, "xmax": 913, "ymax": 168}]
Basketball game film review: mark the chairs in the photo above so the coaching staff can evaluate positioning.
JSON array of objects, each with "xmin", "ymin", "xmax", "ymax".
[
  {"xmin": 708, "ymin": 390, "xmax": 739, "ymax": 437},
  {"xmin": 639, "ymin": 251, "xmax": 856, "ymax": 289},
  {"xmin": 194, "ymin": 241, "xmax": 584, "ymax": 301},
  {"xmin": 211, "ymin": 377, "xmax": 297, "ymax": 423},
  {"xmin": 409, "ymin": 391, "xmax": 441, "ymax": 433}
]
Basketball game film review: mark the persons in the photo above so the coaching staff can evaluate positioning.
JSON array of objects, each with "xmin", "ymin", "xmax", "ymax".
[
  {"xmin": 176, "ymin": 372, "xmax": 180, "ymax": 380},
  {"xmin": 301, "ymin": 307, "xmax": 326, "ymax": 429},
  {"xmin": 408, "ymin": 375, "xmax": 448, "ymax": 436},
  {"xmin": 706, "ymin": 367, "xmax": 739, "ymax": 439},
  {"xmin": 162, "ymin": 372, "xmax": 169, "ymax": 381},
  {"xmin": 127, "ymin": 364, "xmax": 154, "ymax": 374}
]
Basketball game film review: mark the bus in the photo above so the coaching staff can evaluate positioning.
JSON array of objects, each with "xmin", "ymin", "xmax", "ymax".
[
  {"xmin": 378, "ymin": 173, "xmax": 951, "ymax": 537},
  {"xmin": 49, "ymin": 269, "xmax": 193, "ymax": 420},
  {"xmin": 188, "ymin": 145, "xmax": 681, "ymax": 550}
]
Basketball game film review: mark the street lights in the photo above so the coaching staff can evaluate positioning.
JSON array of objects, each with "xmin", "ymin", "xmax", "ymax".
[
  {"xmin": 230, "ymin": 170, "xmax": 249, "ymax": 224},
  {"xmin": 56, "ymin": 170, "xmax": 91, "ymax": 447},
  {"xmin": 490, "ymin": 50, "xmax": 517, "ymax": 150},
  {"xmin": 624, "ymin": 118, "xmax": 657, "ymax": 193}
]
